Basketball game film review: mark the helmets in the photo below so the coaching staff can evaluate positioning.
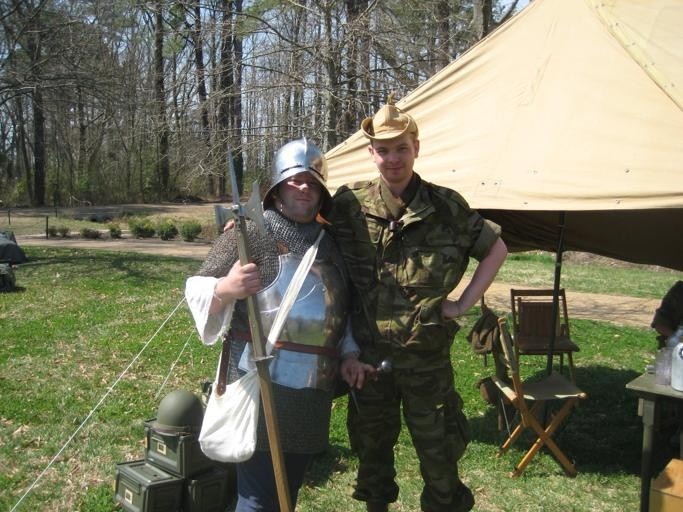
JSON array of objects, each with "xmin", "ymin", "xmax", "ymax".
[
  {"xmin": 262, "ymin": 137, "xmax": 331, "ymax": 210},
  {"xmin": 152, "ymin": 390, "xmax": 203, "ymax": 437}
]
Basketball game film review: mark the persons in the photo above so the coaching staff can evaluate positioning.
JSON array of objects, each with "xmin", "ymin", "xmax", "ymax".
[
  {"xmin": 182, "ymin": 138, "xmax": 375, "ymax": 512},
  {"xmin": 222, "ymin": 96, "xmax": 508, "ymax": 512},
  {"xmin": 651, "ymin": 279, "xmax": 682, "ymax": 340}
]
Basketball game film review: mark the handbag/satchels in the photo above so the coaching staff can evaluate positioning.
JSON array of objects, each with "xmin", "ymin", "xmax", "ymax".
[{"xmin": 197, "ymin": 369, "xmax": 262, "ymax": 463}]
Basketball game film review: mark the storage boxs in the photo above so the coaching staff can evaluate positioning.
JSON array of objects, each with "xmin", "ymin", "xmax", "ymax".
[{"xmin": 111, "ymin": 418, "xmax": 230, "ymax": 512}]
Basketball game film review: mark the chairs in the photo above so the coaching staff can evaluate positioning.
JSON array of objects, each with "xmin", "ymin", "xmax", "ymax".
[
  {"xmin": 479, "ymin": 294, "xmax": 588, "ymax": 479},
  {"xmin": 510, "ymin": 287, "xmax": 581, "ymax": 415}
]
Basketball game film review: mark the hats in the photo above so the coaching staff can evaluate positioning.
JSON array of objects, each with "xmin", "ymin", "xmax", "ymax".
[{"xmin": 360, "ymin": 91, "xmax": 419, "ymax": 141}]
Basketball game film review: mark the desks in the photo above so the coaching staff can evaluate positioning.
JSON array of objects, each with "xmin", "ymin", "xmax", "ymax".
[{"xmin": 625, "ymin": 371, "xmax": 683, "ymax": 512}]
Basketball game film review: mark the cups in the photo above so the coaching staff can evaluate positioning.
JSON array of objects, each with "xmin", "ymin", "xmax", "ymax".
[{"xmin": 654, "ymin": 346, "xmax": 672, "ymax": 386}]
